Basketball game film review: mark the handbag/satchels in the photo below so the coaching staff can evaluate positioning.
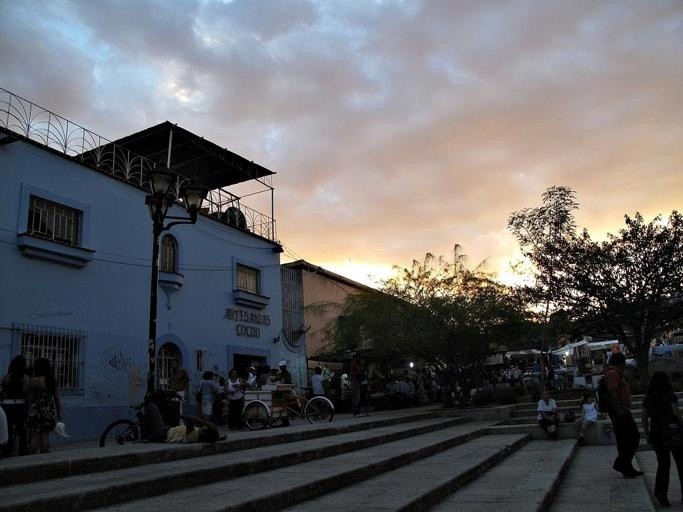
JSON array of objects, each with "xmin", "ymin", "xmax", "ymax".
[{"xmin": 556, "ymin": 411, "xmax": 575, "ymax": 423}]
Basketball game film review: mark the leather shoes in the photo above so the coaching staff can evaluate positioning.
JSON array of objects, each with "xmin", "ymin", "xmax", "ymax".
[{"xmin": 613, "ymin": 462, "xmax": 643, "ymax": 479}]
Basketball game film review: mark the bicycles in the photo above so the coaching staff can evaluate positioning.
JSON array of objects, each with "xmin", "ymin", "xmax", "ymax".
[
  {"xmin": 96, "ymin": 388, "xmax": 184, "ymax": 449},
  {"xmin": 552, "ymin": 369, "xmax": 572, "ymax": 392}
]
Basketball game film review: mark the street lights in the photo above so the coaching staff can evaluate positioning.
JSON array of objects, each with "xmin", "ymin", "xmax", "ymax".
[{"xmin": 139, "ymin": 160, "xmax": 210, "ymax": 412}]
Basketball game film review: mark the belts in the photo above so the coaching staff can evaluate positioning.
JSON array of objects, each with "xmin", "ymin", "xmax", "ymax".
[{"xmin": 2, "ymin": 399, "xmax": 25, "ymax": 404}]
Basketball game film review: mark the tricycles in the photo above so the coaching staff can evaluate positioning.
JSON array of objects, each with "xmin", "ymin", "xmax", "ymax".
[{"xmin": 236, "ymin": 380, "xmax": 338, "ymax": 432}]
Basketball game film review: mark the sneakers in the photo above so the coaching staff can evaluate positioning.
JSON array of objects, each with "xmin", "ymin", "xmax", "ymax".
[{"xmin": 653, "ymin": 493, "xmax": 670, "ymax": 507}]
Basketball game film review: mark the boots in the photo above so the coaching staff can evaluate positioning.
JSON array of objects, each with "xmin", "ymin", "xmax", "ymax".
[{"xmin": 54, "ymin": 422, "xmax": 70, "ymax": 438}]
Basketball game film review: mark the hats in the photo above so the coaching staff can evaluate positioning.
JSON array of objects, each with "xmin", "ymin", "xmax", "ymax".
[{"xmin": 279, "ymin": 361, "xmax": 287, "ymax": 366}]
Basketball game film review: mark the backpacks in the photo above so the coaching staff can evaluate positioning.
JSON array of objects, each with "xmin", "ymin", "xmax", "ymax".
[{"xmin": 599, "ymin": 368, "xmax": 622, "ymax": 412}]
